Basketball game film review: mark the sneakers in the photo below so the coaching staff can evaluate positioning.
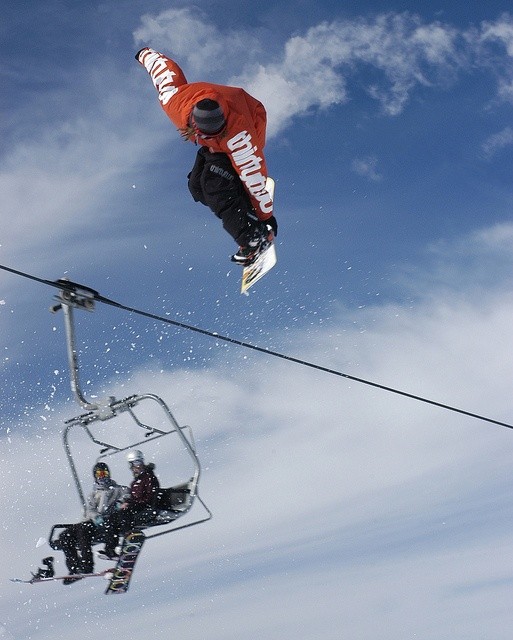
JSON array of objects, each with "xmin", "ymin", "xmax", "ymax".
[
  {"xmin": 70, "ymin": 558, "xmax": 92, "ymax": 574},
  {"xmin": 96, "ymin": 549, "xmax": 122, "ymax": 561},
  {"xmin": 231, "ymin": 228, "xmax": 271, "ymax": 266},
  {"xmin": 63, "ymin": 572, "xmax": 84, "ymax": 584}
]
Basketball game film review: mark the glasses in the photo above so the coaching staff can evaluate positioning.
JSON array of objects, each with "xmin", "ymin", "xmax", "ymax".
[
  {"xmin": 95, "ymin": 471, "xmax": 109, "ymax": 478},
  {"xmin": 128, "ymin": 462, "xmax": 141, "ymax": 469}
]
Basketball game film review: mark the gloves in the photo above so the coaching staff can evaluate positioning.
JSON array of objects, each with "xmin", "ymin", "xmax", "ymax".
[
  {"xmin": 114, "ymin": 499, "xmax": 125, "ymax": 511},
  {"xmin": 95, "ymin": 516, "xmax": 104, "ymax": 526},
  {"xmin": 263, "ymin": 215, "xmax": 277, "ymax": 237}
]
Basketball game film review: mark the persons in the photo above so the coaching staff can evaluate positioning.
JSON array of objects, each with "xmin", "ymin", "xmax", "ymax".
[
  {"xmin": 57, "ymin": 463, "xmax": 125, "ymax": 584},
  {"xmin": 98, "ymin": 450, "xmax": 160, "ymax": 560},
  {"xmin": 139, "ymin": 48, "xmax": 277, "ymax": 266}
]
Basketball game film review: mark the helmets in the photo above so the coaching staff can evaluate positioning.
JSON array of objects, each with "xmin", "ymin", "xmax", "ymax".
[
  {"xmin": 93, "ymin": 462, "xmax": 109, "ymax": 480},
  {"xmin": 127, "ymin": 450, "xmax": 145, "ymax": 468}
]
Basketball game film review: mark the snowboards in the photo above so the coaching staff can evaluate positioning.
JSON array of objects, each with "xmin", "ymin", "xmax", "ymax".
[
  {"xmin": 104, "ymin": 531, "xmax": 145, "ymax": 595},
  {"xmin": 241, "ymin": 177, "xmax": 278, "ymax": 294},
  {"xmin": 9, "ymin": 569, "xmax": 115, "ymax": 582}
]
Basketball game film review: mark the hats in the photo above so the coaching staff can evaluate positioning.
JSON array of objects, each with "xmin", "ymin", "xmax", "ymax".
[{"xmin": 193, "ymin": 99, "xmax": 226, "ymax": 137}]
